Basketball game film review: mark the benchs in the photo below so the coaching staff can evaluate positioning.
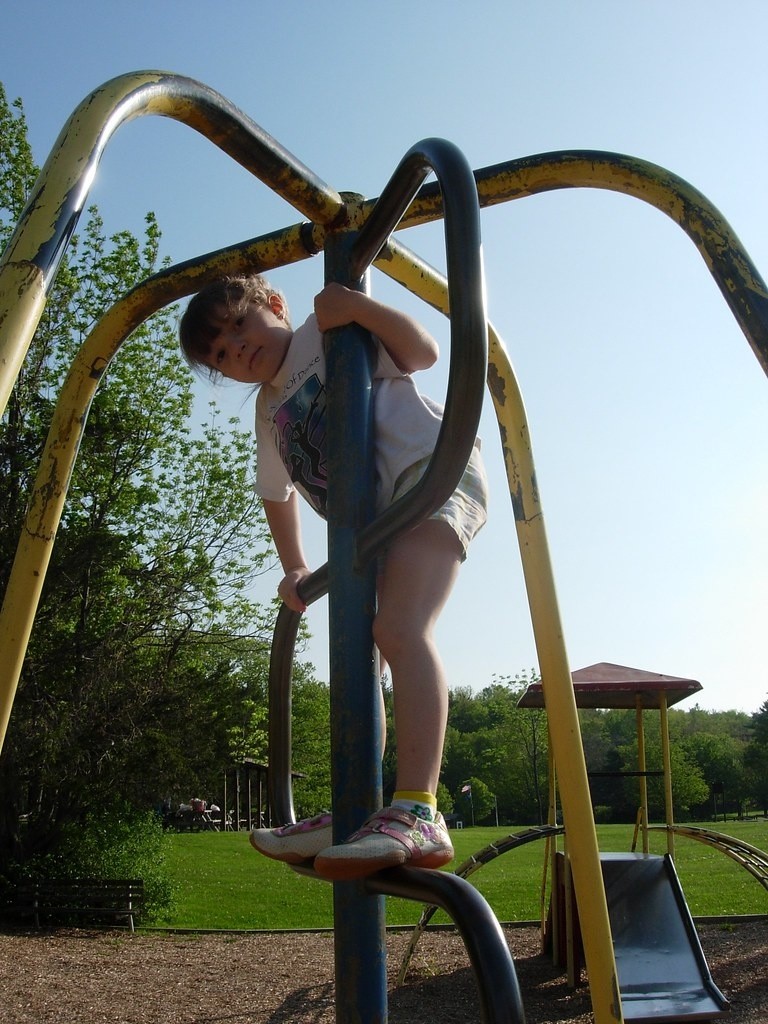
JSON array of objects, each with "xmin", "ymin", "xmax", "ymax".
[
  {"xmin": 0, "ymin": 879, "xmax": 144, "ymax": 933},
  {"xmin": 190, "ymin": 810, "xmax": 266, "ymax": 831}
]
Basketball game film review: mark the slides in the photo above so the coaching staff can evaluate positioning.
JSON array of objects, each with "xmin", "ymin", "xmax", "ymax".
[{"xmin": 578, "ymin": 853, "xmax": 733, "ymax": 1023}]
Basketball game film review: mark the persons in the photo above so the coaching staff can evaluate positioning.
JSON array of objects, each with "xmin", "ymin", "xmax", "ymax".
[{"xmin": 180, "ymin": 278, "xmax": 489, "ymax": 879}]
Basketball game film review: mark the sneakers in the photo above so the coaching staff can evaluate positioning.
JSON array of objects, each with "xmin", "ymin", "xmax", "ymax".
[
  {"xmin": 250, "ymin": 814, "xmax": 335, "ymax": 865},
  {"xmin": 314, "ymin": 804, "xmax": 454, "ymax": 881}
]
